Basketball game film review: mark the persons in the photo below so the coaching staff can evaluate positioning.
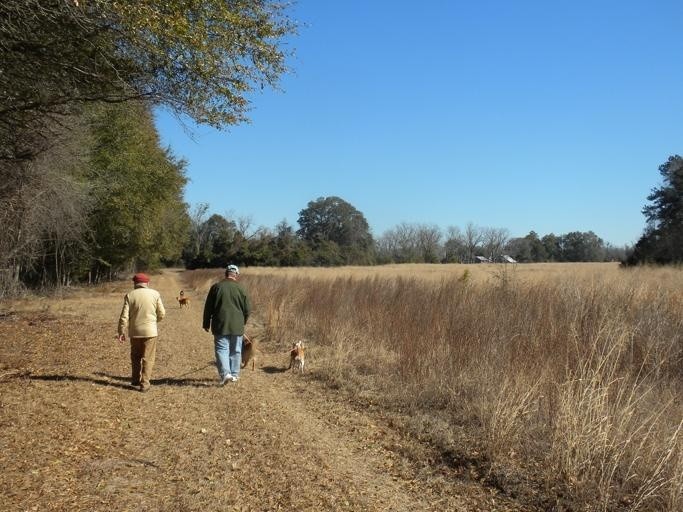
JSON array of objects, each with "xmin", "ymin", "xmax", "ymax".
[
  {"xmin": 203, "ymin": 265, "xmax": 251, "ymax": 386},
  {"xmin": 118, "ymin": 273, "xmax": 167, "ymax": 392}
]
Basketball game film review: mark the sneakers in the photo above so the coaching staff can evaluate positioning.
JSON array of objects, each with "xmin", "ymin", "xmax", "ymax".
[{"xmin": 219, "ymin": 374, "xmax": 237, "ymax": 387}]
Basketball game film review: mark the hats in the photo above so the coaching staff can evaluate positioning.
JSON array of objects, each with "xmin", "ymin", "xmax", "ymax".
[
  {"xmin": 132, "ymin": 273, "xmax": 148, "ymax": 283},
  {"xmin": 226, "ymin": 265, "xmax": 240, "ymax": 275}
]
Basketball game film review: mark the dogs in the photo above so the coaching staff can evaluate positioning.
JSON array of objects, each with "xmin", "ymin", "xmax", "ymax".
[
  {"xmin": 289, "ymin": 340, "xmax": 304, "ymax": 374},
  {"xmin": 176, "ymin": 297, "xmax": 190, "ymax": 310},
  {"xmin": 179, "ymin": 290, "xmax": 184, "ymax": 298},
  {"xmin": 241, "ymin": 336, "xmax": 256, "ymax": 371}
]
